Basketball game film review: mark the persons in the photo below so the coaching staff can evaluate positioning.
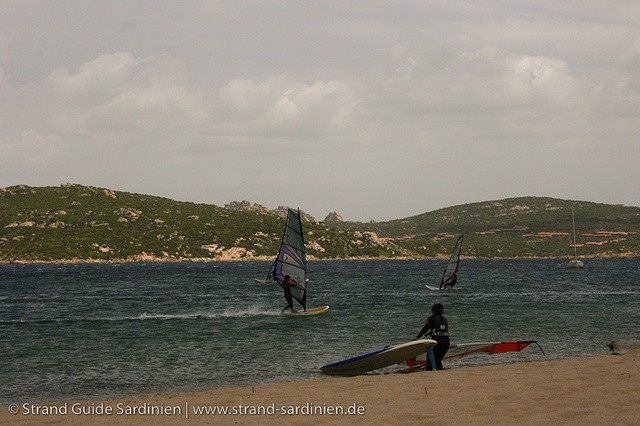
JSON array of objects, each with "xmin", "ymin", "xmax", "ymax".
[
  {"xmin": 414, "ymin": 303, "xmax": 450, "ymax": 370},
  {"xmin": 442, "ymin": 269, "xmax": 459, "ymax": 290},
  {"xmin": 281, "ymin": 274, "xmax": 299, "ymax": 315}
]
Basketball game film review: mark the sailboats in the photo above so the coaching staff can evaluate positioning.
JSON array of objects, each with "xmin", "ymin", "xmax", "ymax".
[{"xmin": 568, "ymin": 207, "xmax": 584, "ymax": 267}]
